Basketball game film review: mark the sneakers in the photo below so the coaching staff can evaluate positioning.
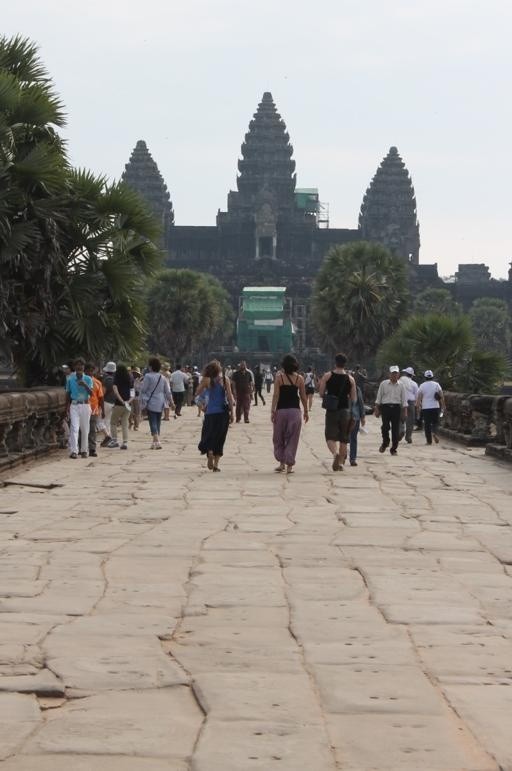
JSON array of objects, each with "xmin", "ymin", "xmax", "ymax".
[
  {"xmin": 397, "ymin": 431, "xmax": 412, "ymax": 443},
  {"xmin": 332, "ymin": 453, "xmax": 341, "ymax": 471},
  {"xmin": 100, "ymin": 435, "xmax": 118, "ymax": 448},
  {"xmin": 379, "ymin": 443, "xmax": 397, "ymax": 455},
  {"xmin": 151, "ymin": 442, "xmax": 162, "ymax": 449},
  {"xmin": 71, "ymin": 449, "xmax": 98, "ymax": 459},
  {"xmin": 119, "ymin": 441, "xmax": 128, "ymax": 449},
  {"xmin": 350, "ymin": 462, "xmax": 358, "ymax": 466},
  {"xmin": 338, "ymin": 464, "xmax": 344, "ymax": 471},
  {"xmin": 433, "ymin": 433, "xmax": 440, "ymax": 443},
  {"xmin": 235, "ymin": 418, "xmax": 251, "ymax": 424}
]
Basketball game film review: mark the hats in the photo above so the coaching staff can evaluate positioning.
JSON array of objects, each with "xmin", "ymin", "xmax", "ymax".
[
  {"xmin": 402, "ymin": 366, "xmax": 416, "ymax": 377},
  {"xmin": 389, "ymin": 365, "xmax": 400, "ymax": 373},
  {"xmin": 102, "ymin": 362, "xmax": 117, "ymax": 373},
  {"xmin": 423, "ymin": 369, "xmax": 434, "ymax": 378},
  {"xmin": 131, "ymin": 367, "xmax": 141, "ymax": 375}
]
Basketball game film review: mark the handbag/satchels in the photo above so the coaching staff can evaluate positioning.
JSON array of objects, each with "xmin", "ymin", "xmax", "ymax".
[
  {"xmin": 434, "ymin": 391, "xmax": 441, "ymax": 401},
  {"xmin": 223, "ymin": 374, "xmax": 236, "ymax": 409},
  {"xmin": 193, "ymin": 387, "xmax": 210, "ymax": 412},
  {"xmin": 141, "ymin": 409, "xmax": 148, "ymax": 416},
  {"xmin": 322, "ymin": 392, "xmax": 340, "ymax": 411}
]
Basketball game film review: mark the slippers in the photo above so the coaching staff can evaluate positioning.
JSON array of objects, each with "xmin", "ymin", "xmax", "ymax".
[
  {"xmin": 287, "ymin": 468, "xmax": 294, "ymax": 473},
  {"xmin": 274, "ymin": 466, "xmax": 286, "ymax": 473}
]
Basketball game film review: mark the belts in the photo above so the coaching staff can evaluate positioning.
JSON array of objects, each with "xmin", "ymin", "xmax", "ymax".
[{"xmin": 71, "ymin": 400, "xmax": 88, "ymax": 405}]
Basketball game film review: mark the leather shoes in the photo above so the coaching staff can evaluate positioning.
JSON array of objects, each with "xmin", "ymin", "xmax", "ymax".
[
  {"xmin": 212, "ymin": 466, "xmax": 221, "ymax": 473},
  {"xmin": 207, "ymin": 454, "xmax": 215, "ymax": 469}
]
